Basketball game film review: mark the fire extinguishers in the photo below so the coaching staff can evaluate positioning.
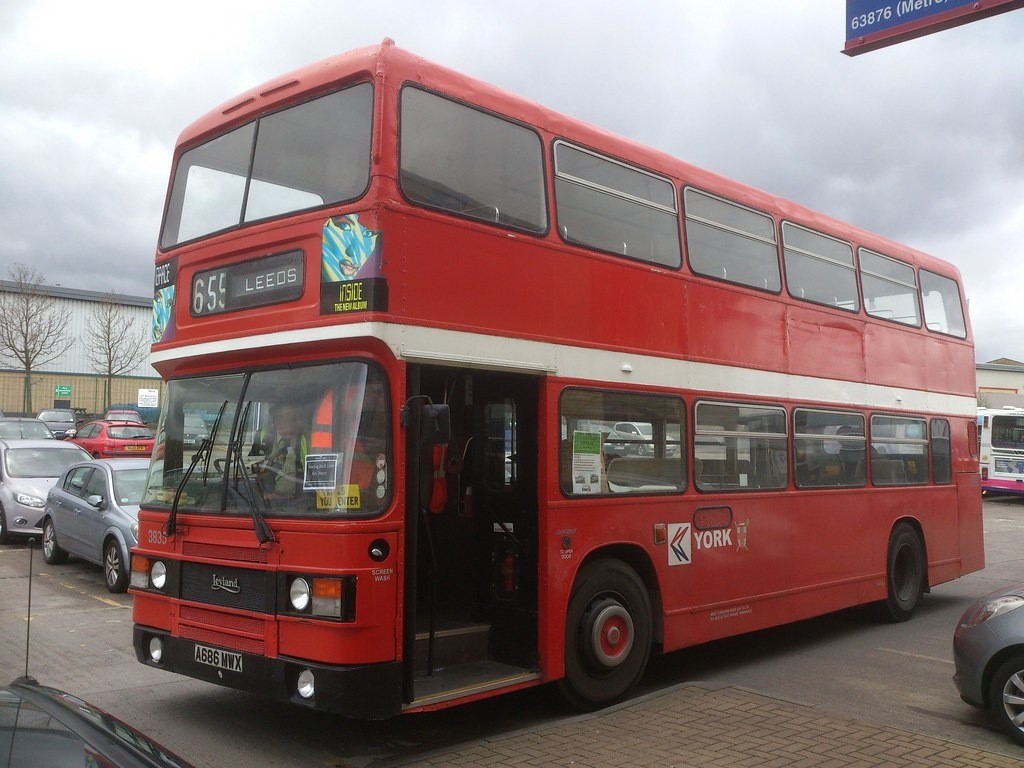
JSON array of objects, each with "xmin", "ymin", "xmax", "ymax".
[{"xmin": 503, "ymin": 544, "xmax": 516, "ymax": 592}]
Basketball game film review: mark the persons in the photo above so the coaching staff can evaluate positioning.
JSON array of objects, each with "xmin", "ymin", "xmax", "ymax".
[
  {"xmin": 311, "ymin": 384, "xmax": 465, "ymax": 518},
  {"xmin": 249, "ymin": 404, "xmax": 309, "ymax": 508},
  {"xmin": 836, "ymin": 427, "xmax": 879, "ymax": 485}
]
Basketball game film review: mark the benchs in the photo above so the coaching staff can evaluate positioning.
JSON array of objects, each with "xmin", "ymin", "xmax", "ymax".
[{"xmin": 606, "ymin": 458, "xmax": 908, "ymax": 487}]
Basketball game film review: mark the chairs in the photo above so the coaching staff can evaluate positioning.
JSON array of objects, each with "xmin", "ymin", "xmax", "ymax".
[{"xmin": 460, "ymin": 205, "xmax": 943, "ymax": 332}]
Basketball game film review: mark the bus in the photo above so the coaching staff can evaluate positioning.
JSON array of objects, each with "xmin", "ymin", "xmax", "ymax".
[
  {"xmin": 122, "ymin": 39, "xmax": 988, "ymax": 724},
  {"xmin": 973, "ymin": 406, "xmax": 1024, "ymax": 493}
]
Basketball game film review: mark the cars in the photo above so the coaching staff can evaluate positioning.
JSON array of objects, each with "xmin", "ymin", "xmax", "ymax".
[
  {"xmin": 40, "ymin": 458, "xmax": 208, "ymax": 596},
  {"xmin": 0, "ymin": 417, "xmax": 55, "ymax": 443},
  {"xmin": 36, "ymin": 409, "xmax": 86, "ymax": 442},
  {"xmin": 0, "ymin": 439, "xmax": 98, "ymax": 544},
  {"xmin": 63, "ymin": 420, "xmax": 164, "ymax": 459},
  {"xmin": 105, "ymin": 410, "xmax": 144, "ymax": 427},
  {"xmin": 181, "ymin": 414, "xmax": 210, "ymax": 450},
  {"xmin": 951, "ymin": 581, "xmax": 1024, "ymax": 744}
]
